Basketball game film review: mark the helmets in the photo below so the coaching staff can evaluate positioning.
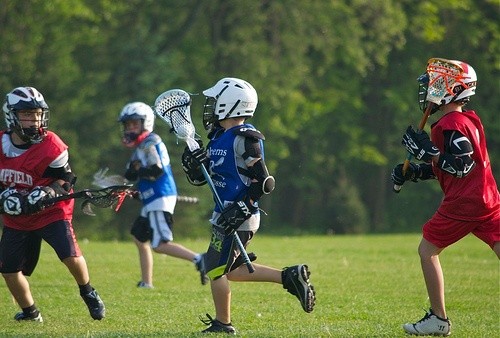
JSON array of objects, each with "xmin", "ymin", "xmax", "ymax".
[
  {"xmin": 2, "ymin": 87, "xmax": 50, "ymax": 144},
  {"xmin": 117, "ymin": 102, "xmax": 154, "ymax": 147},
  {"xmin": 203, "ymin": 77, "xmax": 259, "ymax": 128},
  {"xmin": 417, "ymin": 58, "xmax": 477, "ymax": 117}
]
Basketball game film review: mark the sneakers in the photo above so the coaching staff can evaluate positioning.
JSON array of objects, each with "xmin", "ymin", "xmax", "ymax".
[
  {"xmin": 403, "ymin": 307, "xmax": 451, "ymax": 337},
  {"xmin": 14, "ymin": 311, "xmax": 44, "ymax": 323},
  {"xmin": 281, "ymin": 264, "xmax": 317, "ymax": 313},
  {"xmin": 137, "ymin": 281, "xmax": 155, "ymax": 289},
  {"xmin": 194, "ymin": 253, "xmax": 209, "ymax": 285},
  {"xmin": 199, "ymin": 314, "xmax": 237, "ymax": 336},
  {"xmin": 80, "ymin": 287, "xmax": 105, "ymax": 320}
]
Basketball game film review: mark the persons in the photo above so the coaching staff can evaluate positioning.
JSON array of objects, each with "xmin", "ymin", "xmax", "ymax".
[
  {"xmin": 117, "ymin": 102, "xmax": 209, "ymax": 288},
  {"xmin": 182, "ymin": 77, "xmax": 316, "ymax": 334},
  {"xmin": 390, "ymin": 57, "xmax": 500, "ymax": 336},
  {"xmin": 0, "ymin": 87, "xmax": 106, "ymax": 322}
]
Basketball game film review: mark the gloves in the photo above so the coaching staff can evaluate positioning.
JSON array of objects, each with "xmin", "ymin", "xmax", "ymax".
[
  {"xmin": 401, "ymin": 124, "xmax": 440, "ymax": 163},
  {"xmin": 217, "ymin": 195, "xmax": 259, "ymax": 237},
  {"xmin": 0, "ymin": 181, "xmax": 68, "ymax": 217},
  {"xmin": 182, "ymin": 139, "xmax": 210, "ymax": 176},
  {"xmin": 125, "ymin": 161, "xmax": 142, "ymax": 181},
  {"xmin": 392, "ymin": 163, "xmax": 435, "ymax": 186}
]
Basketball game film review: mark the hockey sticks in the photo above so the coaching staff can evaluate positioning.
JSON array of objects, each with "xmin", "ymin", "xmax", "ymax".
[
  {"xmin": 393, "ymin": 58, "xmax": 465, "ymax": 193},
  {"xmin": 90, "ymin": 172, "xmax": 198, "ymax": 204},
  {"xmin": 38, "ymin": 184, "xmax": 136, "ymax": 210},
  {"xmin": 152, "ymin": 88, "xmax": 255, "ymax": 274}
]
